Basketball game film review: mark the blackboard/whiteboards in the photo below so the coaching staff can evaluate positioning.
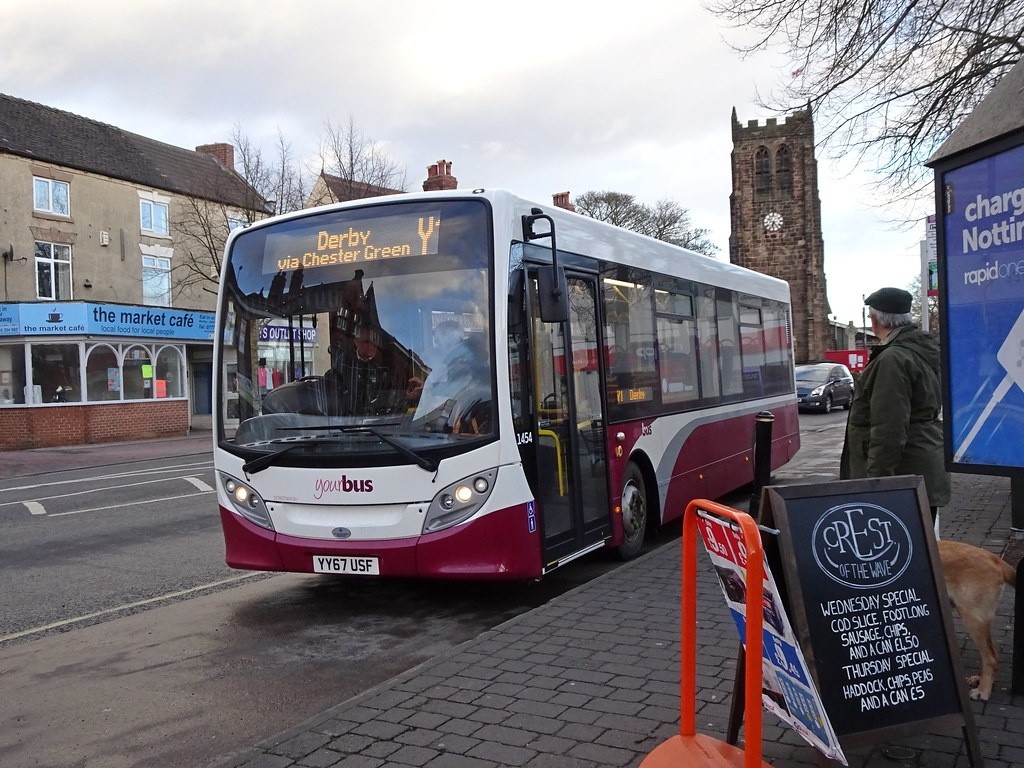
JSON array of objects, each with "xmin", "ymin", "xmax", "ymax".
[{"xmin": 735, "ymin": 473, "xmax": 973, "ymax": 759}]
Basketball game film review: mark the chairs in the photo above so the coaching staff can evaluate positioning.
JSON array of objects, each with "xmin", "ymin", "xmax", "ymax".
[{"xmin": 518, "ymin": 324, "xmax": 795, "ymax": 408}]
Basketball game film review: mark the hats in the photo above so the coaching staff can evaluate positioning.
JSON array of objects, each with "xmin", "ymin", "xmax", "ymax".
[{"xmin": 865, "ymin": 287, "xmax": 913, "ymax": 314}]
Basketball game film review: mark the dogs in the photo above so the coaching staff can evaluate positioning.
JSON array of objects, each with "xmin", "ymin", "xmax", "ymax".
[{"xmin": 936, "ymin": 537, "xmax": 1015, "ymax": 700}]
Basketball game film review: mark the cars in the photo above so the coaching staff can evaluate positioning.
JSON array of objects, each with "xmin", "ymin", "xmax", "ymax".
[{"xmin": 795, "ymin": 363, "xmax": 854, "ymax": 414}]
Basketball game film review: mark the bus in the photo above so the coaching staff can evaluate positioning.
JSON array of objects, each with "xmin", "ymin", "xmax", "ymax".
[{"xmin": 209, "ymin": 189, "xmax": 800, "ymax": 586}]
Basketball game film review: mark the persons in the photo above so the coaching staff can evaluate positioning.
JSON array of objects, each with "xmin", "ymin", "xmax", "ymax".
[
  {"xmin": 321, "ymin": 315, "xmax": 525, "ymax": 433},
  {"xmin": 839, "ymin": 287, "xmax": 951, "ymax": 534}
]
